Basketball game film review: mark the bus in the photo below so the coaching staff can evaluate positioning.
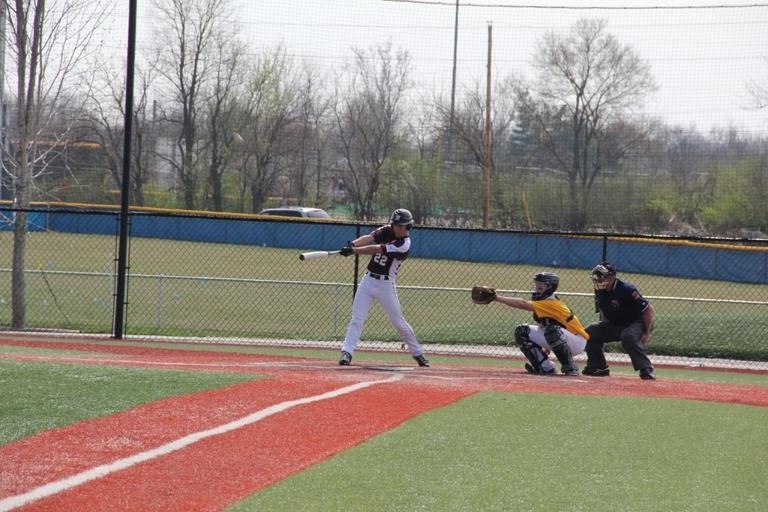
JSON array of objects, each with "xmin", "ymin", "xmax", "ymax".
[{"xmin": 3, "ymin": 139, "xmax": 113, "ymax": 203}]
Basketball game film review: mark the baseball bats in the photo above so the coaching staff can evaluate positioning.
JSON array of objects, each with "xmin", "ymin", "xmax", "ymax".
[{"xmin": 300, "ymin": 250, "xmax": 342, "ymax": 260}]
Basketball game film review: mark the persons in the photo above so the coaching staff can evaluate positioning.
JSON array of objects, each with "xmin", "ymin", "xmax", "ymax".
[
  {"xmin": 582, "ymin": 262, "xmax": 656, "ymax": 380},
  {"xmin": 337, "ymin": 207, "xmax": 430, "ymax": 365},
  {"xmin": 482, "ymin": 270, "xmax": 590, "ymax": 377}
]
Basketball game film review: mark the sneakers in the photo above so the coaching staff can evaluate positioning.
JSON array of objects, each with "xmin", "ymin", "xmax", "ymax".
[
  {"xmin": 339, "ymin": 351, "xmax": 352, "ymax": 365},
  {"xmin": 413, "ymin": 354, "xmax": 429, "ymax": 366},
  {"xmin": 528, "ymin": 367, "xmax": 656, "ymax": 379}
]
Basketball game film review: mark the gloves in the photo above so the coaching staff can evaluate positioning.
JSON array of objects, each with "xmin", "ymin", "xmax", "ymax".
[
  {"xmin": 347, "ymin": 241, "xmax": 354, "ymax": 247},
  {"xmin": 339, "ymin": 247, "xmax": 354, "ymax": 256}
]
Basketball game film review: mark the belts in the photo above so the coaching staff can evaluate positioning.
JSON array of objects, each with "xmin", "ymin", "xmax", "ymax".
[{"xmin": 366, "ymin": 272, "xmax": 388, "ymax": 280}]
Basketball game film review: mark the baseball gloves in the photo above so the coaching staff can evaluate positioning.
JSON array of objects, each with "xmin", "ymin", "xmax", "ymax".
[{"xmin": 472, "ymin": 286, "xmax": 496, "ymax": 304}]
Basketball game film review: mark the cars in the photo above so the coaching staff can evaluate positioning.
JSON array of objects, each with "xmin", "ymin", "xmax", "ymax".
[{"xmin": 257, "ymin": 206, "xmax": 331, "ymax": 220}]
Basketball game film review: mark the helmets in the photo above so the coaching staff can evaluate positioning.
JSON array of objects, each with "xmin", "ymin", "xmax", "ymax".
[
  {"xmin": 532, "ymin": 272, "xmax": 559, "ymax": 300},
  {"xmin": 590, "ymin": 262, "xmax": 616, "ymax": 291},
  {"xmin": 390, "ymin": 209, "xmax": 413, "ymax": 228}
]
